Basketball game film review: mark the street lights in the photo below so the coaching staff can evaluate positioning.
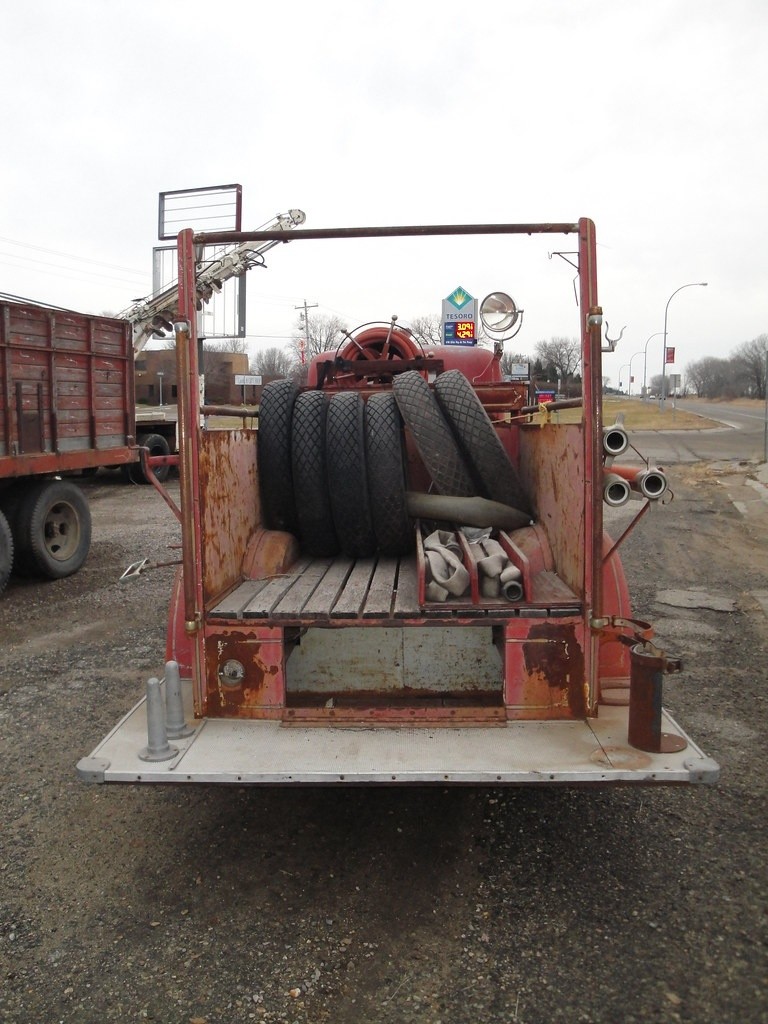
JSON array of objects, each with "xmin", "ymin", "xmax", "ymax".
[{"xmin": 660, "ymin": 282, "xmax": 709, "ymax": 408}]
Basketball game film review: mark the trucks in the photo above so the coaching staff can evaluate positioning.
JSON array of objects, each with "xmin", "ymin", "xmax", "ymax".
[
  {"xmin": 0, "ymin": 295, "xmax": 140, "ymax": 593},
  {"xmin": 82, "ymin": 344, "xmax": 250, "ymax": 487},
  {"xmin": 66, "ymin": 216, "xmax": 723, "ymax": 796}
]
